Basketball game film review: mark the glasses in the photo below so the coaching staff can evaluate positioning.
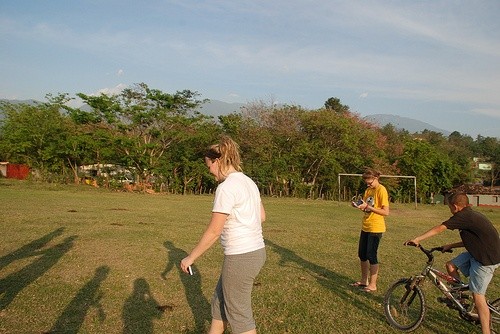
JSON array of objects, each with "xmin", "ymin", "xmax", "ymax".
[{"xmin": 368, "ymin": 178, "xmax": 375, "ymax": 185}]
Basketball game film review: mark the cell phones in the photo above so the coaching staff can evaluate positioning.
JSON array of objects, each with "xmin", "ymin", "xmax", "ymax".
[{"xmin": 187, "ymin": 266, "xmax": 193, "ymax": 275}]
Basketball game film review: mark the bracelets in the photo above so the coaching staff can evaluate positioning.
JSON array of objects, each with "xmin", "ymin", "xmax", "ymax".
[{"xmin": 364, "ymin": 203, "xmax": 368, "ymax": 209}]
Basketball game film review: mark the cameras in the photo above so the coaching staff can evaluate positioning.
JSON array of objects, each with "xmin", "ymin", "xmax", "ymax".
[{"xmin": 358, "ymin": 199, "xmax": 362, "ymax": 205}]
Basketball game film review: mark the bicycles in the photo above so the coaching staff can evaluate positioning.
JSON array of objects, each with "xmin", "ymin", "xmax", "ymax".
[{"xmin": 383, "ymin": 242, "xmax": 500, "ymax": 334}]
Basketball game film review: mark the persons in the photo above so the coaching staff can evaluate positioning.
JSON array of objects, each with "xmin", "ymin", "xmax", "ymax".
[
  {"xmin": 180, "ymin": 135, "xmax": 267, "ymax": 334},
  {"xmin": 402, "ymin": 191, "xmax": 500, "ymax": 334},
  {"xmin": 351, "ymin": 169, "xmax": 390, "ymax": 292}
]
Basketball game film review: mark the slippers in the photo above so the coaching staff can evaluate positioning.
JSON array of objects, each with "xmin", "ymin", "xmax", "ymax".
[
  {"xmin": 352, "ymin": 281, "xmax": 367, "ymax": 287},
  {"xmin": 363, "ymin": 288, "xmax": 377, "ymax": 292}
]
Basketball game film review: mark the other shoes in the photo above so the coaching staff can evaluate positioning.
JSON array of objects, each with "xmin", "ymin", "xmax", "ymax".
[{"xmin": 438, "ymin": 296, "xmax": 451, "ymax": 303}]
